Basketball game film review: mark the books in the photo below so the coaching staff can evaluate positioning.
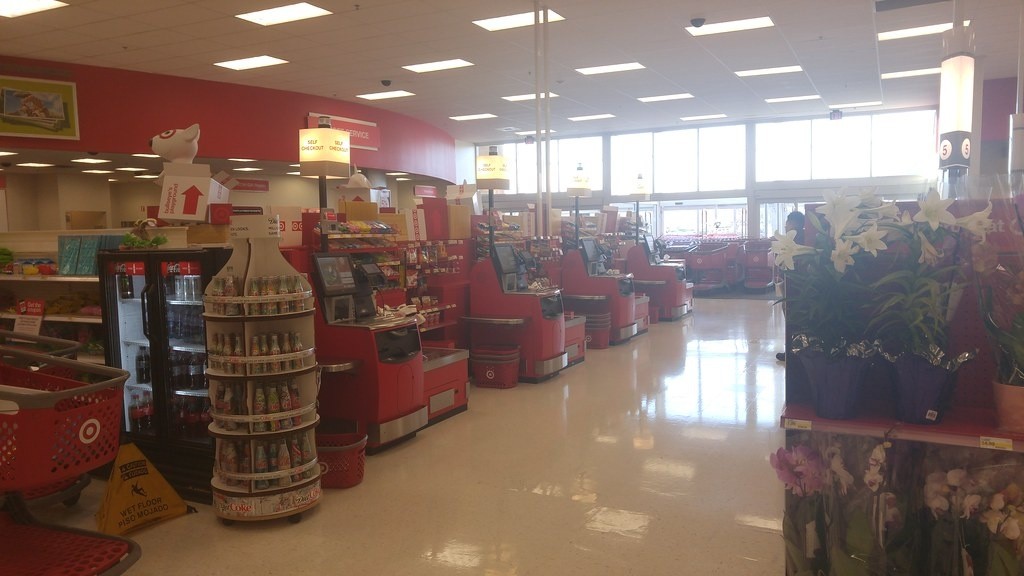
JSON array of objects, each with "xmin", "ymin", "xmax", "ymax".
[{"xmin": 57, "ymin": 235, "xmax": 120, "ymax": 276}]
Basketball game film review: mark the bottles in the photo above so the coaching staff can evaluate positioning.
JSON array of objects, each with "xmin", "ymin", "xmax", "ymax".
[
  {"xmin": 208, "ymin": 276, "xmax": 313, "ymax": 489},
  {"xmin": 130, "ymin": 305, "xmax": 211, "ymax": 435}
]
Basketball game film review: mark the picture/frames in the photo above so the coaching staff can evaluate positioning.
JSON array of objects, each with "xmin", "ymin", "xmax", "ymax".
[{"xmin": 0, "ymin": 75, "xmax": 80, "ymax": 141}]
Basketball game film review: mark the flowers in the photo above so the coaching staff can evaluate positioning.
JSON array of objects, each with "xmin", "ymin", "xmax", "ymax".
[
  {"xmin": 770, "ymin": 438, "xmax": 1024, "ymax": 576},
  {"xmin": 865, "ymin": 187, "xmax": 994, "ymax": 362},
  {"xmin": 768, "ymin": 187, "xmax": 903, "ymax": 360}
]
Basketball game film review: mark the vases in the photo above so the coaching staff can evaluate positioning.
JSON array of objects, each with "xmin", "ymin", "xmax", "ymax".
[
  {"xmin": 795, "ymin": 349, "xmax": 871, "ymax": 420},
  {"xmin": 891, "ymin": 360, "xmax": 953, "ymax": 423}
]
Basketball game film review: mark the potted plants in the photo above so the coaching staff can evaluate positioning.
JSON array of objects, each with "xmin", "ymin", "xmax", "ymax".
[{"xmin": 976, "ymin": 276, "xmax": 1024, "ymax": 434}]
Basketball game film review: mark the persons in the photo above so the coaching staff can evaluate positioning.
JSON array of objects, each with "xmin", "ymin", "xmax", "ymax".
[{"xmin": 776, "ymin": 210, "xmax": 805, "ymax": 362}]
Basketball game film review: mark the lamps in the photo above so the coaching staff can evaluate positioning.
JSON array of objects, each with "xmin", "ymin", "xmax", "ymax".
[
  {"xmin": 477, "ymin": 155, "xmax": 510, "ymax": 190},
  {"xmin": 298, "ymin": 128, "xmax": 351, "ymax": 180},
  {"xmin": 939, "ymin": 52, "xmax": 974, "ymax": 170}
]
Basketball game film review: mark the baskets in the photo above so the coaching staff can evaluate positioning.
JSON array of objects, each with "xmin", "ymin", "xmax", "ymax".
[
  {"xmin": 584, "ymin": 311, "xmax": 611, "ymax": 348},
  {"xmin": 469, "ymin": 343, "xmax": 521, "ymax": 389},
  {"xmin": 315, "ymin": 432, "xmax": 368, "ymax": 487}
]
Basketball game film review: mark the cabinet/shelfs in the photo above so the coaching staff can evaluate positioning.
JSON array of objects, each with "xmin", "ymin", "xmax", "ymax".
[
  {"xmin": 200, "ymin": 215, "xmax": 323, "ymax": 527},
  {"xmin": 325, "ymin": 226, "xmax": 598, "ymax": 333},
  {"xmin": 0, "ymin": 273, "xmax": 105, "ymax": 365}
]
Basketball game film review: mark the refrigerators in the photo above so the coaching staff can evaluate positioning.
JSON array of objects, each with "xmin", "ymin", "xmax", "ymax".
[{"xmin": 98, "ymin": 246, "xmax": 233, "ymax": 504}]
